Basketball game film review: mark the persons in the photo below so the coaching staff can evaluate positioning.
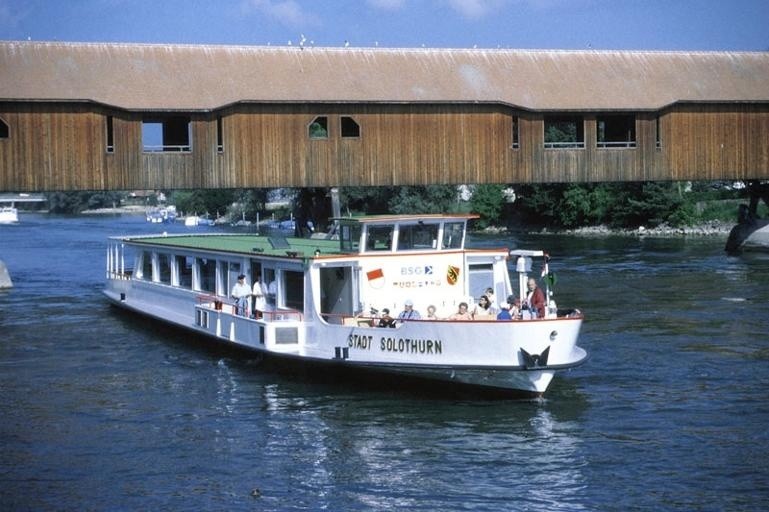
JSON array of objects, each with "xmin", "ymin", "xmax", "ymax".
[
  {"xmin": 371, "ymin": 278, "xmax": 545, "ymax": 332},
  {"xmin": 232, "ymin": 274, "xmax": 252, "ymax": 316},
  {"xmin": 252, "ymin": 272, "xmax": 268, "ymax": 317}
]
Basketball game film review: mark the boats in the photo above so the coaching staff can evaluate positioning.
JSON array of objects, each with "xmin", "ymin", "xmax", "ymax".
[
  {"xmin": 188, "ymin": 346, "xmax": 592, "ymax": 512},
  {"xmin": 0, "ymin": 205, "xmax": 20, "ymax": 227},
  {"xmin": 142, "ymin": 207, "xmax": 315, "ymax": 231},
  {"xmin": 98, "ymin": 211, "xmax": 592, "ymax": 397}
]
